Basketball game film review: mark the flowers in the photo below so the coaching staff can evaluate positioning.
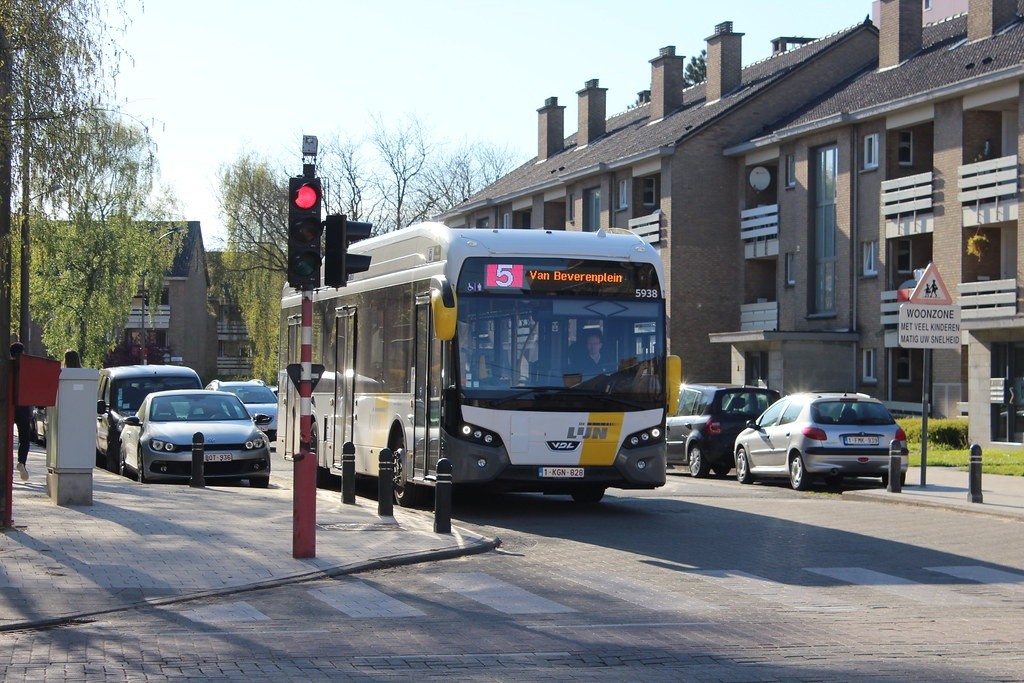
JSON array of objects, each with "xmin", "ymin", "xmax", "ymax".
[{"xmin": 966, "ymin": 234, "xmax": 991, "ymax": 261}]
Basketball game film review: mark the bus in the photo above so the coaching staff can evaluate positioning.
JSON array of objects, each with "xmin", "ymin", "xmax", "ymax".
[{"xmin": 276, "ymin": 221, "xmax": 681, "ymax": 506}]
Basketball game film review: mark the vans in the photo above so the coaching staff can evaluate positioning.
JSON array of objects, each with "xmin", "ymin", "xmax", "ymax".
[{"xmin": 96, "ymin": 365, "xmax": 203, "ymax": 472}]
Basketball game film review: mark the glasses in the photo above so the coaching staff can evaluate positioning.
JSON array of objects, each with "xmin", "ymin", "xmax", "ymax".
[{"xmin": 587, "ymin": 342, "xmax": 600, "ymax": 345}]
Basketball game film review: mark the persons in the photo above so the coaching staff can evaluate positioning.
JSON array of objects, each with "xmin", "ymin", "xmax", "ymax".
[
  {"xmin": 10, "ymin": 343, "xmax": 32, "ymax": 481},
  {"xmin": 575, "ymin": 330, "xmax": 616, "ymax": 376},
  {"xmin": 59, "ymin": 347, "xmax": 86, "ymax": 367}
]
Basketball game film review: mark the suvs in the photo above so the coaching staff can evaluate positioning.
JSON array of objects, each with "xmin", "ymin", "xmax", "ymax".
[
  {"xmin": 665, "ymin": 383, "xmax": 781, "ymax": 478},
  {"xmin": 205, "ymin": 379, "xmax": 278, "ymax": 442}
]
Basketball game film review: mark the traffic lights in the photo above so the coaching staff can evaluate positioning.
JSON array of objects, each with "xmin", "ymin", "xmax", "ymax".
[
  {"xmin": 287, "ymin": 177, "xmax": 324, "ymax": 291},
  {"xmin": 326, "ymin": 215, "xmax": 372, "ymax": 288}
]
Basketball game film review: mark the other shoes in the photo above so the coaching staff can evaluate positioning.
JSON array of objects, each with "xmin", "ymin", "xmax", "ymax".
[{"xmin": 17, "ymin": 463, "xmax": 29, "ymax": 480}]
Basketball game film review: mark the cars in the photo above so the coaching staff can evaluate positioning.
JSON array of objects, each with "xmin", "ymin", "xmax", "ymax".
[
  {"xmin": 733, "ymin": 392, "xmax": 909, "ymax": 489},
  {"xmin": 119, "ymin": 389, "xmax": 271, "ymax": 488}
]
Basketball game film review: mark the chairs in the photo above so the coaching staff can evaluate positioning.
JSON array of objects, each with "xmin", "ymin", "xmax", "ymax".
[
  {"xmin": 155, "ymin": 403, "xmax": 177, "ymax": 420},
  {"xmin": 188, "ymin": 405, "xmax": 209, "ymax": 419},
  {"xmin": 561, "ymin": 342, "xmax": 582, "ymax": 385},
  {"xmin": 838, "ymin": 408, "xmax": 857, "ymax": 422}
]
